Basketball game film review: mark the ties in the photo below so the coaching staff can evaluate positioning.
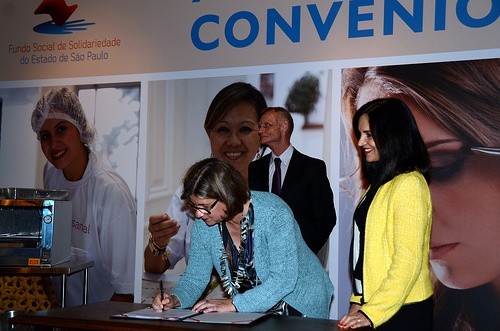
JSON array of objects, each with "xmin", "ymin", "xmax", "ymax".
[{"xmin": 271, "ymin": 158, "xmax": 282, "ymax": 196}]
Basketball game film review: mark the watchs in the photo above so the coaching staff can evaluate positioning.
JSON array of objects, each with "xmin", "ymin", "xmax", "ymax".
[{"xmin": 149, "ymin": 239, "xmax": 165, "ymax": 256}]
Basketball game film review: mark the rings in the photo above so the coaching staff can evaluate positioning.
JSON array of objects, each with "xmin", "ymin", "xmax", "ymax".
[{"xmin": 357, "ymin": 319, "xmax": 361, "ymax": 323}]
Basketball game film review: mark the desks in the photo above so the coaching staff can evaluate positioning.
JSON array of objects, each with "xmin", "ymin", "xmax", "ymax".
[
  {"xmin": 0, "ymin": 255, "xmax": 94, "ymax": 308},
  {"xmin": 7, "ymin": 303, "xmax": 370, "ymax": 331}
]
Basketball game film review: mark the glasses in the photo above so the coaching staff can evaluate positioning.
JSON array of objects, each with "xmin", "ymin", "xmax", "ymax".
[{"xmin": 187, "ymin": 198, "xmax": 220, "ymax": 215}]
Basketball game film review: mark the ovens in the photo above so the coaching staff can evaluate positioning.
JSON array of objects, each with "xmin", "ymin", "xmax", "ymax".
[{"xmin": 0, "ymin": 199, "xmax": 72, "ymax": 266}]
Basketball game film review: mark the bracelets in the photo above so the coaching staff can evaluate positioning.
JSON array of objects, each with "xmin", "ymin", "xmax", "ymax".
[{"xmin": 152, "ymin": 237, "xmax": 167, "ymax": 249}]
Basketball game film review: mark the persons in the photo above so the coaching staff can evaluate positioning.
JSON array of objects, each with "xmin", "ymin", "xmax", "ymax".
[
  {"xmin": 144, "ymin": 82, "xmax": 269, "ymax": 275},
  {"xmin": 337, "ymin": 97, "xmax": 435, "ymax": 331},
  {"xmin": 31, "ymin": 88, "xmax": 137, "ymax": 310},
  {"xmin": 341, "ymin": 58, "xmax": 500, "ymax": 331},
  {"xmin": 152, "ymin": 157, "xmax": 335, "ymax": 319},
  {"xmin": 248, "ymin": 106, "xmax": 337, "ymax": 255}
]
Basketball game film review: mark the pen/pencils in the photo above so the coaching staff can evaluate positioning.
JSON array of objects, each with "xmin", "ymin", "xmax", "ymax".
[{"xmin": 160, "ymin": 280, "xmax": 164, "ymax": 312}]
